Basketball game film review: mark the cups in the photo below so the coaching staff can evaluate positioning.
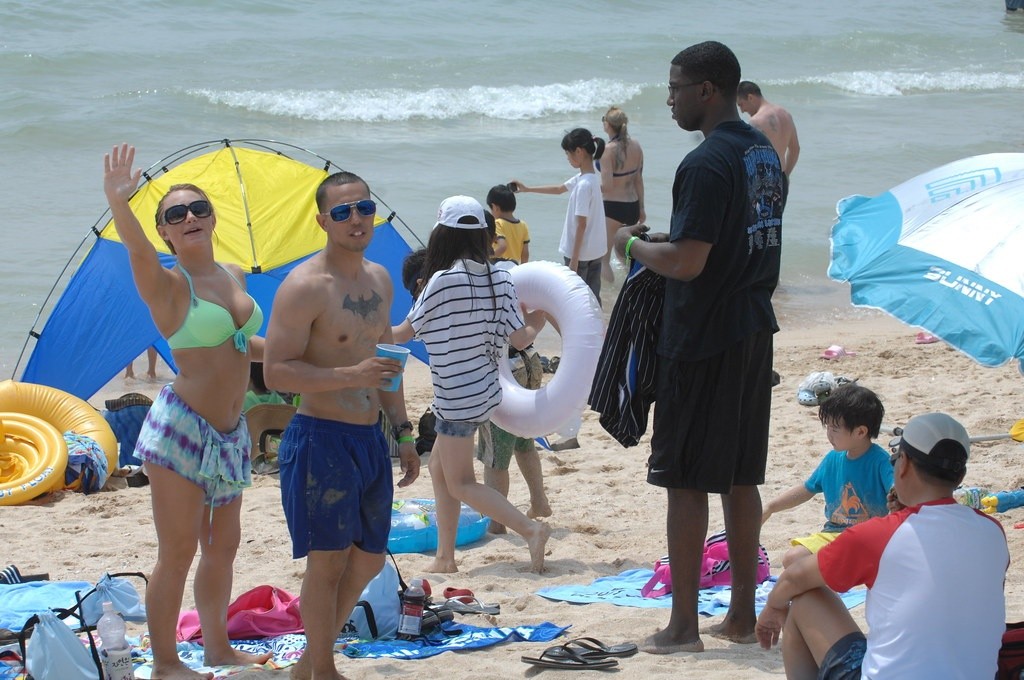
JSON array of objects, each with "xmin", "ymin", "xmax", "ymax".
[{"xmin": 375, "ymin": 343, "xmax": 410, "ymax": 392}]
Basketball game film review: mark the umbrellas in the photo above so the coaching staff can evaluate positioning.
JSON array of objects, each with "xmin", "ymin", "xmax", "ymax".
[{"xmin": 825, "ymin": 156, "xmax": 1024, "ymax": 382}]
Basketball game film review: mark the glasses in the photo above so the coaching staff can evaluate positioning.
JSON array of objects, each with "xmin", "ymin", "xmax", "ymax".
[
  {"xmin": 160, "ymin": 200, "xmax": 212, "ymax": 225},
  {"xmin": 602, "ymin": 115, "xmax": 605, "ymax": 122},
  {"xmin": 890, "ymin": 453, "xmax": 904, "ymax": 468},
  {"xmin": 322, "ymin": 199, "xmax": 376, "ymax": 223},
  {"xmin": 668, "ymin": 83, "xmax": 703, "ymax": 98}
]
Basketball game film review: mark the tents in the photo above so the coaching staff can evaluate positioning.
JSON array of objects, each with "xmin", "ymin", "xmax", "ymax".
[{"xmin": 13, "ymin": 133, "xmax": 431, "ymax": 448}]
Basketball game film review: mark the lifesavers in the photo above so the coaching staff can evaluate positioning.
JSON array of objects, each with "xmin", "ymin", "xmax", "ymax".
[
  {"xmin": 0, "ymin": 379, "xmax": 119, "ymax": 506},
  {"xmin": 490, "ymin": 260, "xmax": 607, "ymax": 439},
  {"xmin": 386, "ymin": 498, "xmax": 492, "ymax": 553}
]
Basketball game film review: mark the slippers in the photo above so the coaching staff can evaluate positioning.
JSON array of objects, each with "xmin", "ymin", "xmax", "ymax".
[
  {"xmin": 821, "ymin": 345, "xmax": 858, "ymax": 360},
  {"xmin": 915, "ymin": 331, "xmax": 937, "ymax": 344},
  {"xmin": 521, "ymin": 645, "xmax": 619, "ymax": 669},
  {"xmin": 543, "ymin": 637, "xmax": 638, "ymax": 658},
  {"xmin": 434, "ymin": 595, "xmax": 500, "ymax": 615}
]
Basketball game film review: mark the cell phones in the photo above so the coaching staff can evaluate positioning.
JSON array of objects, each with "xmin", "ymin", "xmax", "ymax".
[{"xmin": 507, "ymin": 183, "xmax": 517, "ymax": 192}]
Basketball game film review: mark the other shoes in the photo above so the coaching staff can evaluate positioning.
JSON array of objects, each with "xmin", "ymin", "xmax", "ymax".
[
  {"xmin": 540, "ymin": 356, "xmax": 561, "ymax": 375},
  {"xmin": 443, "ymin": 587, "xmax": 473, "ymax": 603}
]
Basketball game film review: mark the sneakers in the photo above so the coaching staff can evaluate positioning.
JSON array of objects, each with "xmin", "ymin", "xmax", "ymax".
[{"xmin": 422, "ymin": 604, "xmax": 454, "ymax": 630}]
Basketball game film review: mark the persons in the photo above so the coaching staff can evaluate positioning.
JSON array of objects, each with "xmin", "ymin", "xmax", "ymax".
[
  {"xmin": 126, "ymin": 344, "xmax": 158, "ymax": 382},
  {"xmin": 754, "ymin": 411, "xmax": 1010, "ymax": 680},
  {"xmin": 263, "ymin": 172, "xmax": 421, "ymax": 680},
  {"xmin": 386, "ymin": 193, "xmax": 551, "ymax": 574},
  {"xmin": 403, "ymin": 246, "xmax": 554, "ymax": 536},
  {"xmin": 104, "ymin": 143, "xmax": 274, "ymax": 680},
  {"xmin": 487, "ymin": 183, "xmax": 531, "ymax": 268},
  {"xmin": 735, "ymin": 78, "xmax": 800, "ymax": 175},
  {"xmin": 598, "ymin": 104, "xmax": 647, "ymax": 282},
  {"xmin": 239, "ymin": 361, "xmax": 287, "ymax": 460},
  {"xmin": 761, "ymin": 383, "xmax": 896, "ymax": 571},
  {"xmin": 610, "ymin": 41, "xmax": 785, "ymax": 658},
  {"xmin": 506, "ymin": 128, "xmax": 608, "ymax": 310}
]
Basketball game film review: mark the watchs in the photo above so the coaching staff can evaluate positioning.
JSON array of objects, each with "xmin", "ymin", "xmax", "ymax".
[{"xmin": 388, "ymin": 421, "xmax": 413, "ymax": 439}]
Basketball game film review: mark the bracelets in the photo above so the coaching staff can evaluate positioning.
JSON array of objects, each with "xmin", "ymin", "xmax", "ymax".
[
  {"xmin": 624, "ymin": 236, "xmax": 640, "ymax": 268},
  {"xmin": 399, "ymin": 436, "xmax": 416, "ymax": 443}
]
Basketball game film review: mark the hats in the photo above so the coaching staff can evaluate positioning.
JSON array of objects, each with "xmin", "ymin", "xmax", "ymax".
[
  {"xmin": 432, "ymin": 195, "xmax": 488, "ymax": 229},
  {"xmin": 889, "ymin": 413, "xmax": 971, "ymax": 464}
]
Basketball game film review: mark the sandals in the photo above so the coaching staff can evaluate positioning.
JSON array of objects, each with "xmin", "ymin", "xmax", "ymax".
[{"xmin": 797, "ymin": 372, "xmax": 860, "ymax": 406}]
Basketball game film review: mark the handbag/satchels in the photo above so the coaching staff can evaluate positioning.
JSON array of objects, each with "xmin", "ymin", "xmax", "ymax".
[
  {"xmin": 641, "ymin": 529, "xmax": 771, "ymax": 598},
  {"xmin": 174, "ymin": 583, "xmax": 307, "ymax": 646},
  {"xmin": 18, "ymin": 608, "xmax": 107, "ymax": 680},
  {"xmin": 338, "ymin": 548, "xmax": 408, "ymax": 640},
  {"xmin": 75, "ymin": 572, "xmax": 149, "ymax": 628}
]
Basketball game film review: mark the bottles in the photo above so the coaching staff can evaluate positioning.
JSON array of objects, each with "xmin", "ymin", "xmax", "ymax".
[
  {"xmin": 953, "ymin": 486, "xmax": 993, "ymax": 510},
  {"xmin": 397, "ymin": 578, "xmax": 426, "ymax": 640},
  {"xmin": 97, "ymin": 602, "xmax": 134, "ymax": 680}
]
